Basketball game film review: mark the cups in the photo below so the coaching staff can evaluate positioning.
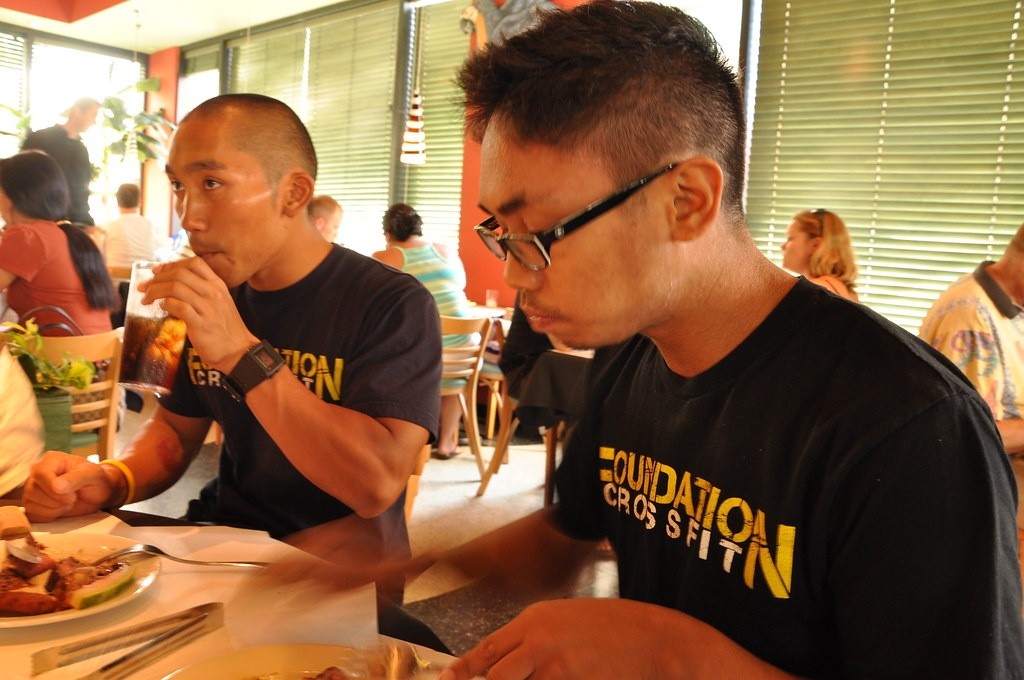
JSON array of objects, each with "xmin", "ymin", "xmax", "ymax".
[
  {"xmin": 118, "ymin": 258, "xmax": 189, "ymax": 395},
  {"xmin": 486, "ymin": 289, "xmax": 499, "ymax": 308}
]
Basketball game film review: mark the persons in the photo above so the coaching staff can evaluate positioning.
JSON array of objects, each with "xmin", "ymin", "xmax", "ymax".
[
  {"xmin": 371, "ymin": 204, "xmax": 482, "ymax": 461},
  {"xmin": 917, "ymin": 223, "xmax": 1024, "ymax": 454},
  {"xmin": 102, "ymin": 183, "xmax": 160, "ymax": 283},
  {"xmin": 232, "ymin": 0, "xmax": 1024, "ymax": 680},
  {"xmin": 0, "ymin": 95, "xmax": 122, "ymax": 506},
  {"xmin": 25, "ymin": 92, "xmax": 443, "ymax": 603},
  {"xmin": 307, "ymin": 194, "xmax": 343, "ymax": 242},
  {"xmin": 780, "ymin": 208, "xmax": 859, "ymax": 304}
]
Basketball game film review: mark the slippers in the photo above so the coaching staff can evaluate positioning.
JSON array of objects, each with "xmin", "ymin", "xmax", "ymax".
[{"xmin": 439, "ymin": 446, "xmax": 460, "ymax": 459}]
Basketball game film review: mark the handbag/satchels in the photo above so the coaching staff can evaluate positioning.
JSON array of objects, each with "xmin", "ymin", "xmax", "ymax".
[{"xmin": 17, "ymin": 305, "xmax": 123, "ymax": 433}]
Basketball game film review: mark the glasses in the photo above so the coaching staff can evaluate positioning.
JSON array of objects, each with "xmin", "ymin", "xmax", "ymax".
[
  {"xmin": 474, "ymin": 162, "xmax": 680, "ymax": 271},
  {"xmin": 810, "ymin": 209, "xmax": 828, "ymax": 238}
]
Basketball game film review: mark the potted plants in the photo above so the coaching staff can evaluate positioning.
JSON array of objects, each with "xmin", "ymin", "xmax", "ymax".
[{"xmin": 2, "ymin": 317, "xmax": 95, "ymax": 454}]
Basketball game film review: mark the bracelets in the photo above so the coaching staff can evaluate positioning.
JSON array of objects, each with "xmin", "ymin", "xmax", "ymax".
[{"xmin": 100, "ymin": 458, "xmax": 136, "ymax": 515}]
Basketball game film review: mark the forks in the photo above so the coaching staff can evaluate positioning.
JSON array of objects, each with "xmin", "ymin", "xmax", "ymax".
[{"xmin": 88, "ymin": 544, "xmax": 273, "ymax": 570}]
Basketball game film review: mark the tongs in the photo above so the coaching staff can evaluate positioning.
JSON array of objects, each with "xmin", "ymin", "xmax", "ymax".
[{"xmin": 30, "ymin": 602, "xmax": 225, "ymax": 680}]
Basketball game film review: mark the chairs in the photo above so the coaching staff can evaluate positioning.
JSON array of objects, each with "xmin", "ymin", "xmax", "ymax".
[
  {"xmin": 0, "ymin": 326, "xmax": 126, "ymax": 464},
  {"xmin": 438, "ymin": 316, "xmax": 567, "ymax": 507}
]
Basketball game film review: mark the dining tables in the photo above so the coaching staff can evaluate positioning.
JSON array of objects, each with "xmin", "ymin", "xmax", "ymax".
[{"xmin": 0, "ymin": 497, "xmax": 486, "ymax": 680}]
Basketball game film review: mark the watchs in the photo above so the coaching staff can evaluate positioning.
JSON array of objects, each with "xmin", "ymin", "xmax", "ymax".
[{"xmin": 220, "ymin": 338, "xmax": 286, "ymax": 404}]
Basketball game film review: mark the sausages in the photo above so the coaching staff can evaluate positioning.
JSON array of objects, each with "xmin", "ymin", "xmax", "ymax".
[{"xmin": 0, "ymin": 592, "xmax": 58, "ymax": 615}]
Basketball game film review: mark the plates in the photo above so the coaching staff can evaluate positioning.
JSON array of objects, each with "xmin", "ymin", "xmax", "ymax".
[
  {"xmin": 0, "ymin": 532, "xmax": 161, "ymax": 627},
  {"xmin": 161, "ymin": 643, "xmax": 375, "ymax": 680}
]
